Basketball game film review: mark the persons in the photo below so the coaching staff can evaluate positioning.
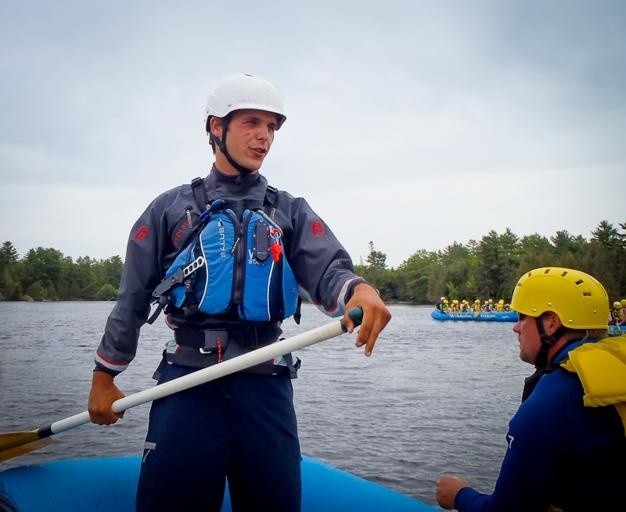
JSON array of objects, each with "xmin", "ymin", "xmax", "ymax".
[
  {"xmin": 609, "ymin": 297, "xmax": 626, "ymax": 330},
  {"xmin": 87, "ymin": 72, "xmax": 394, "ymax": 510},
  {"xmin": 435, "ymin": 295, "xmax": 514, "ymax": 315},
  {"xmin": 433, "ymin": 263, "xmax": 626, "ymax": 512}
]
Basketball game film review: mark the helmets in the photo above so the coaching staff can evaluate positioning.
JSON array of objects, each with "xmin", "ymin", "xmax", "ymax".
[
  {"xmin": 509, "ymin": 266, "xmax": 611, "ymax": 332},
  {"xmin": 203, "ymin": 72, "xmax": 286, "ymax": 134},
  {"xmin": 612, "ymin": 299, "xmax": 626, "ymax": 313},
  {"xmin": 438, "ymin": 295, "xmax": 505, "ymax": 307}
]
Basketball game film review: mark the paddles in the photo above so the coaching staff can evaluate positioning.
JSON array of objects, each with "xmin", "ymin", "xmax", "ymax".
[{"xmin": 0, "ymin": 307, "xmax": 362, "ymax": 461}]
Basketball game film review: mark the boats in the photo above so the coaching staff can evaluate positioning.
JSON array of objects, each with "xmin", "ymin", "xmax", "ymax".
[
  {"xmin": 608, "ymin": 324, "xmax": 626, "ymax": 337},
  {"xmin": 431, "ymin": 307, "xmax": 520, "ymax": 321},
  {"xmin": 0, "ymin": 451, "xmax": 452, "ymax": 512}
]
